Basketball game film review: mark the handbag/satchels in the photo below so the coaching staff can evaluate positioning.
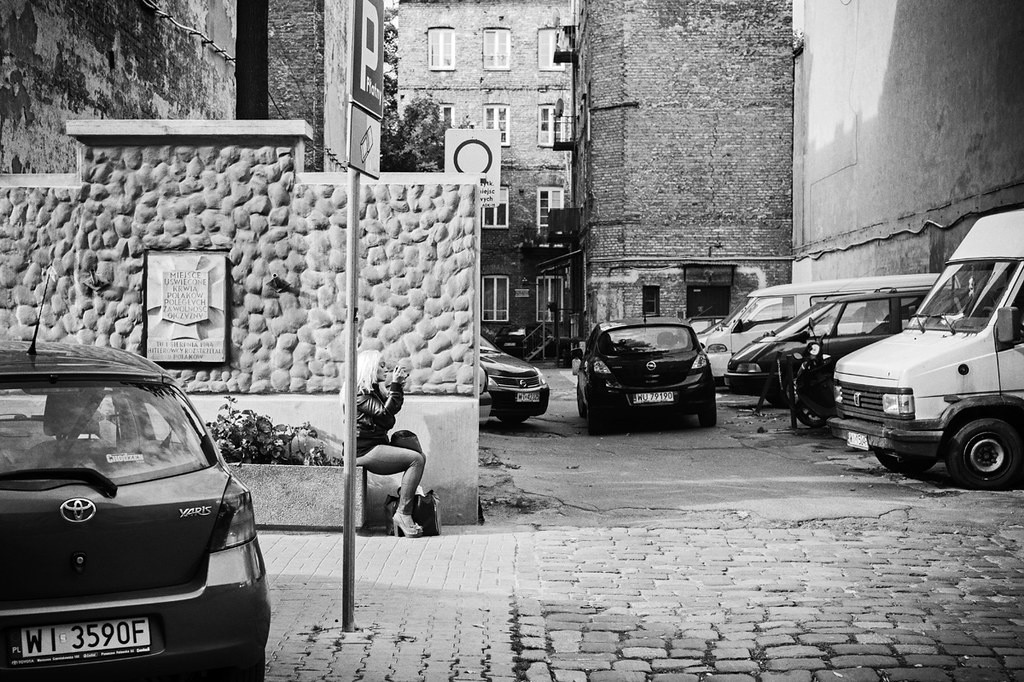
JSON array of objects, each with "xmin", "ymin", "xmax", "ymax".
[{"xmin": 383, "ymin": 487, "xmax": 442, "ymax": 536}]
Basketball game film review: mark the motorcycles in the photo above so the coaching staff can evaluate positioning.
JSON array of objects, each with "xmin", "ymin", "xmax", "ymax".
[{"xmin": 790, "ymin": 320, "xmax": 836, "ymax": 429}]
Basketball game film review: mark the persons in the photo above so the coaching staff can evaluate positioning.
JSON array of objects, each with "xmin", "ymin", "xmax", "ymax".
[{"xmin": 340, "ymin": 348, "xmax": 428, "ymax": 539}]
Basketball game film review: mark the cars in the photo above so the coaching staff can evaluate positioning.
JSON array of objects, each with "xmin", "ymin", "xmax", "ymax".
[
  {"xmin": 696, "ymin": 318, "xmax": 725, "ymax": 346},
  {"xmin": 480, "ymin": 332, "xmax": 550, "ymax": 423},
  {"xmin": 571, "ymin": 314, "xmax": 717, "ymax": 433},
  {"xmin": 0, "ymin": 338, "xmax": 272, "ymax": 682},
  {"xmin": 675, "ymin": 315, "xmax": 726, "ymax": 336}
]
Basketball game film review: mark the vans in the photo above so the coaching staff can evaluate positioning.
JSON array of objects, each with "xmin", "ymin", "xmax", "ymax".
[
  {"xmin": 825, "ymin": 207, "xmax": 1024, "ymax": 489},
  {"xmin": 723, "ymin": 285, "xmax": 933, "ymax": 409},
  {"xmin": 706, "ymin": 273, "xmax": 942, "ymax": 387}
]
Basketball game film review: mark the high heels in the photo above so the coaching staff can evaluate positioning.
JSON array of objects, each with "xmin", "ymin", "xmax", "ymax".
[{"xmin": 392, "ymin": 512, "xmax": 424, "ymax": 538}]
